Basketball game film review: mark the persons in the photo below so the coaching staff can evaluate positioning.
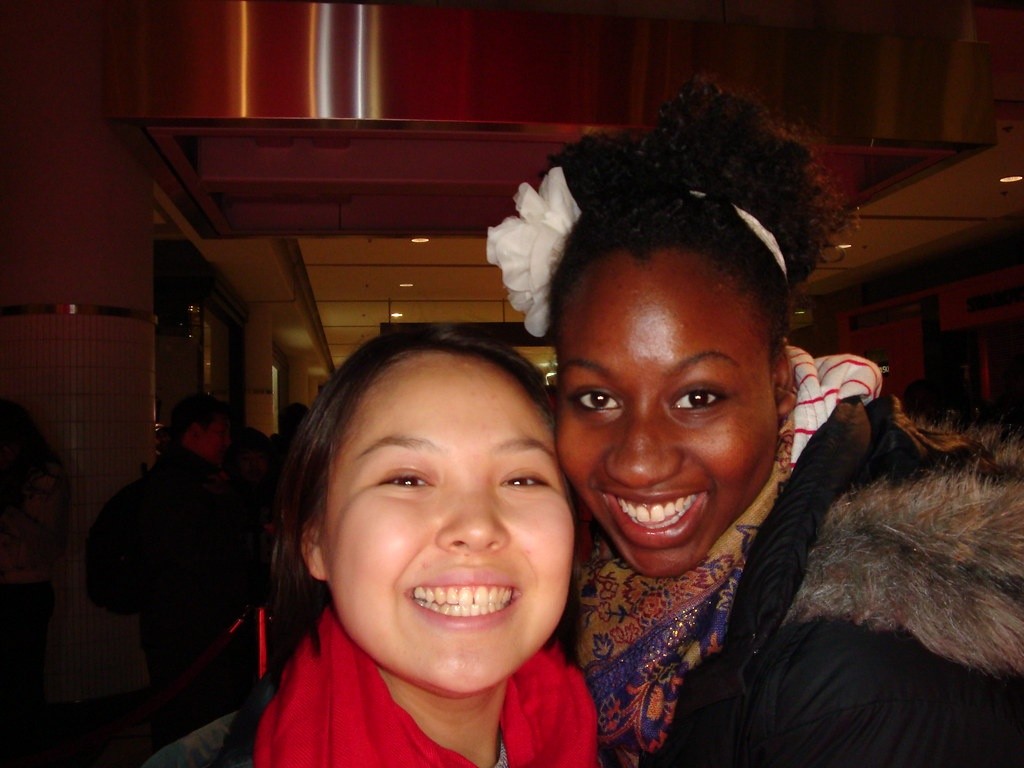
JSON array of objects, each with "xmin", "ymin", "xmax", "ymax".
[
  {"xmin": 131, "ymin": 325, "xmax": 603, "ymax": 768},
  {"xmin": 141, "ymin": 394, "xmax": 308, "ymax": 754},
  {"xmin": 0, "ymin": 398, "xmax": 73, "ymax": 765},
  {"xmin": 487, "ymin": 83, "xmax": 1024, "ymax": 768}
]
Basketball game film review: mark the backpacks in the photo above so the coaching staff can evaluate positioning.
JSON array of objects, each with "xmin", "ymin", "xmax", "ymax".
[{"xmin": 85, "ymin": 467, "xmax": 151, "ymax": 616}]
